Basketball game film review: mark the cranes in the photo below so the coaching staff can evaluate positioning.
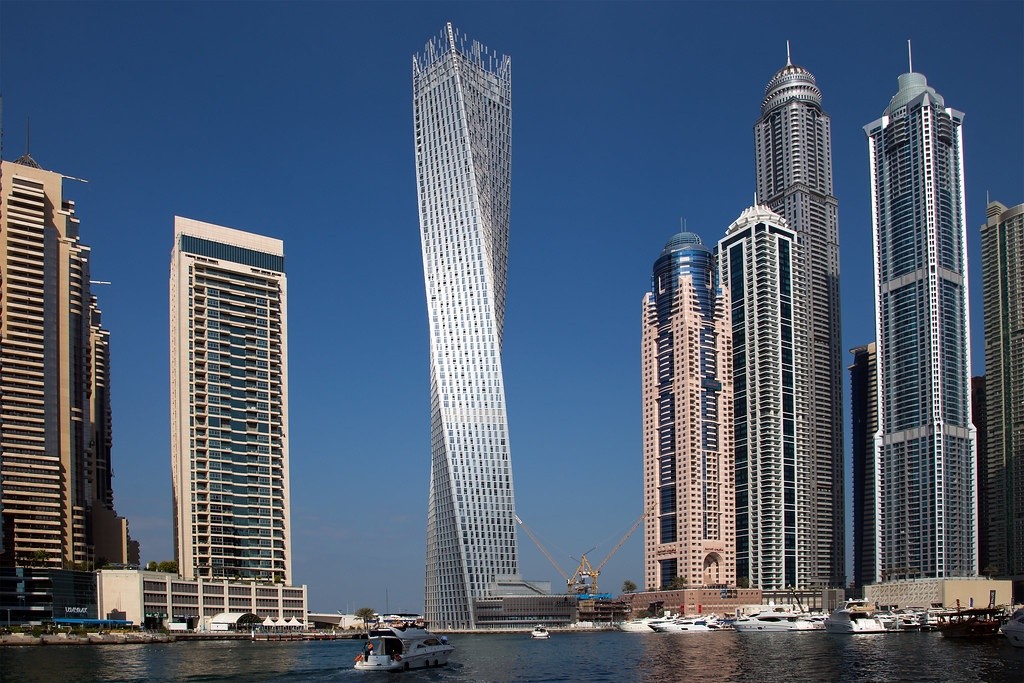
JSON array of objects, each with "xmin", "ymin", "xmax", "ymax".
[{"xmin": 513, "ymin": 499, "xmax": 659, "ymax": 593}]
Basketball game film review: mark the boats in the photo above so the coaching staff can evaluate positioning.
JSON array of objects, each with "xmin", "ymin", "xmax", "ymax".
[
  {"xmin": 531, "ymin": 624, "xmax": 550, "ymax": 641},
  {"xmin": 616, "ymin": 600, "xmax": 1024, "ymax": 652},
  {"xmin": 0, "ymin": 622, "xmax": 178, "ymax": 647},
  {"xmin": 353, "ymin": 620, "xmax": 456, "ymax": 673}
]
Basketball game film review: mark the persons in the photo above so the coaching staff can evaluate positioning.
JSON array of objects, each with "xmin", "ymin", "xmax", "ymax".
[
  {"xmin": 362, "ymin": 641, "xmax": 373, "ymax": 662},
  {"xmin": 402, "ymin": 620, "xmax": 419, "ymax": 632}
]
